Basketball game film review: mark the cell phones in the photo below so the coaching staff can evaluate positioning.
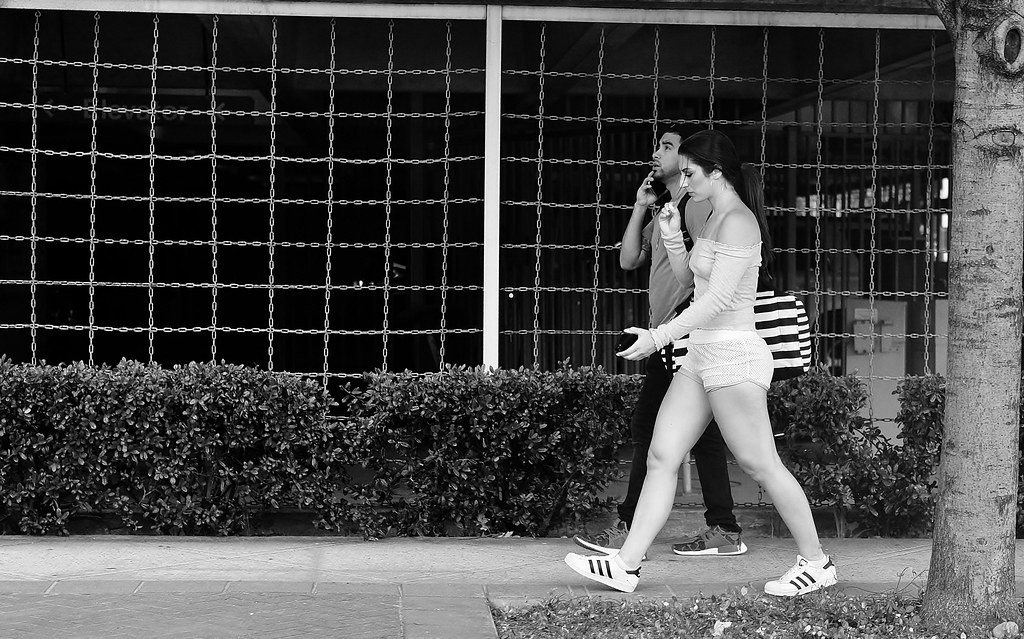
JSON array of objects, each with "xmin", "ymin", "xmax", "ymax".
[
  {"xmin": 614, "ymin": 333, "xmax": 638, "ymax": 351},
  {"xmin": 648, "ymin": 174, "xmax": 666, "ymax": 197}
]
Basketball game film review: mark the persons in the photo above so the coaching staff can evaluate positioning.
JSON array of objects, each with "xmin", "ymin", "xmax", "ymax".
[{"xmin": 564, "ymin": 125, "xmax": 838, "ymax": 596}]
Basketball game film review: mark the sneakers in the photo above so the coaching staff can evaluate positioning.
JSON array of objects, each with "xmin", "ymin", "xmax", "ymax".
[
  {"xmin": 764, "ymin": 552, "xmax": 838, "ymax": 597},
  {"xmin": 565, "ymin": 552, "xmax": 642, "ymax": 593},
  {"xmin": 671, "ymin": 524, "xmax": 748, "ymax": 556},
  {"xmin": 572, "ymin": 517, "xmax": 647, "ymax": 561}
]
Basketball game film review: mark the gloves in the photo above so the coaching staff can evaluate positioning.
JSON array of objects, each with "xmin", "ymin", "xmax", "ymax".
[
  {"xmin": 658, "ymin": 201, "xmax": 681, "ymax": 236},
  {"xmin": 616, "ymin": 326, "xmax": 658, "ymax": 361}
]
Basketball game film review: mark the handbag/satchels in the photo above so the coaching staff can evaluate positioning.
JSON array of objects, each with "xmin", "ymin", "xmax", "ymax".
[{"xmin": 660, "ymin": 287, "xmax": 814, "ymax": 384}]
